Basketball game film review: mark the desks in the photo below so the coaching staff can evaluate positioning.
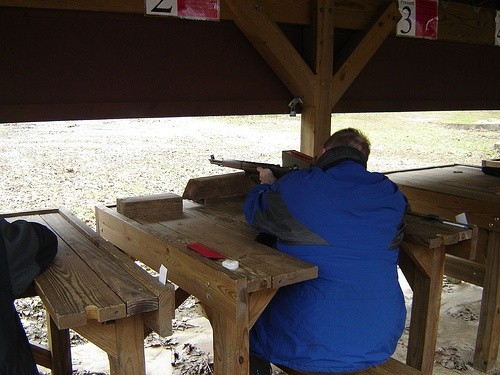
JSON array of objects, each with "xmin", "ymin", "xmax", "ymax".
[{"xmin": 0, "ymin": 164, "xmax": 500, "ymax": 375}]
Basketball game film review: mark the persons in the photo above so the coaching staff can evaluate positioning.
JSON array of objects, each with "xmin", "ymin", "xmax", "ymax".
[
  {"xmin": 242, "ymin": 128, "xmax": 409, "ymax": 375},
  {"xmin": 0, "ymin": 213, "xmax": 58, "ymax": 375}
]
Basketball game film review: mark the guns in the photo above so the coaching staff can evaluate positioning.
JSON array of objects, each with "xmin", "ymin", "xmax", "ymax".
[{"xmin": 208, "ymin": 154, "xmax": 299, "ymax": 185}]
